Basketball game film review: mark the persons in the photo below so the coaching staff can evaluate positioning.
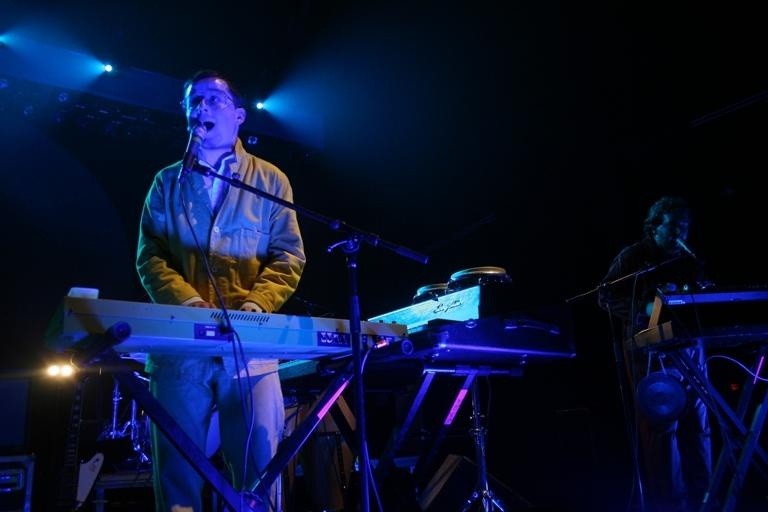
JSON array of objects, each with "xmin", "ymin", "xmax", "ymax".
[
  {"xmin": 598, "ymin": 194, "xmax": 716, "ymax": 511},
  {"xmin": 136, "ymin": 68, "xmax": 307, "ymax": 512}
]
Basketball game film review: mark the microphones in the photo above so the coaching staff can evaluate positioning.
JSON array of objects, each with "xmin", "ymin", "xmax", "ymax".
[
  {"xmin": 676, "ymin": 237, "xmax": 697, "ymax": 258},
  {"xmin": 177, "ymin": 124, "xmax": 207, "ymax": 184}
]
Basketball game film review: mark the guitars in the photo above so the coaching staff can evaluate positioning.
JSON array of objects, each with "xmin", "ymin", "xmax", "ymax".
[{"xmin": 43, "ymin": 371, "xmax": 105, "ymax": 511}]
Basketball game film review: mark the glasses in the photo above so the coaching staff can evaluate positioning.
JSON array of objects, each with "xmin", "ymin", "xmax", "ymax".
[{"xmin": 180, "ymin": 89, "xmax": 235, "ymax": 109}]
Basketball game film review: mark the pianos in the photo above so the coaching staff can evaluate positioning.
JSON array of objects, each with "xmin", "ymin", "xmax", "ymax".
[
  {"xmin": 44, "ymin": 295, "xmax": 406, "ymax": 364},
  {"xmin": 629, "ymin": 286, "xmax": 767, "ymax": 348},
  {"xmin": 363, "ymin": 319, "xmax": 576, "ymax": 365}
]
